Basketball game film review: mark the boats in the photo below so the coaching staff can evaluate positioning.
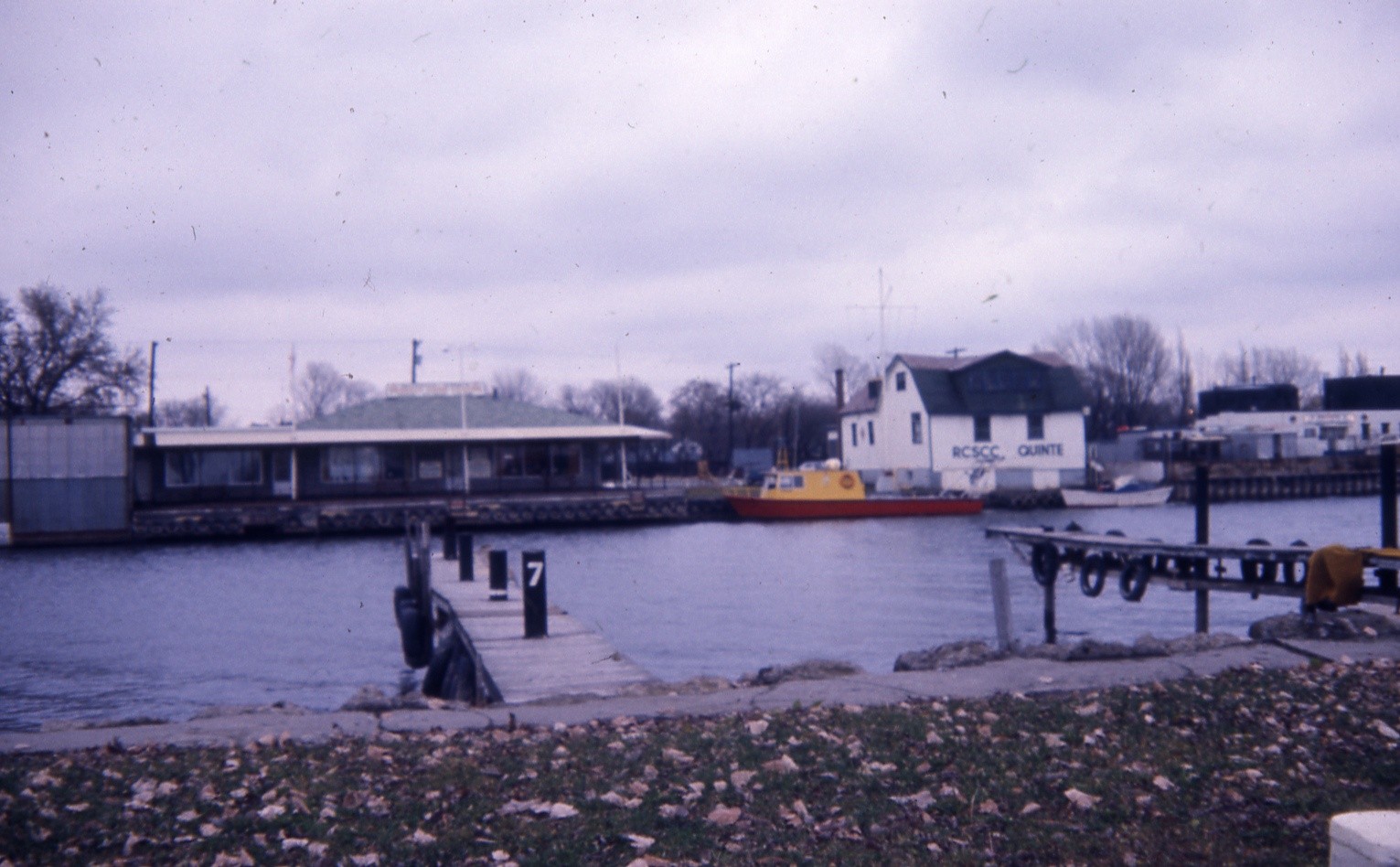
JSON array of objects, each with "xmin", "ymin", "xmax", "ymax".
[
  {"xmin": 721, "ymin": 442, "xmax": 986, "ymax": 518},
  {"xmin": 1061, "ymin": 480, "xmax": 1175, "ymax": 506}
]
